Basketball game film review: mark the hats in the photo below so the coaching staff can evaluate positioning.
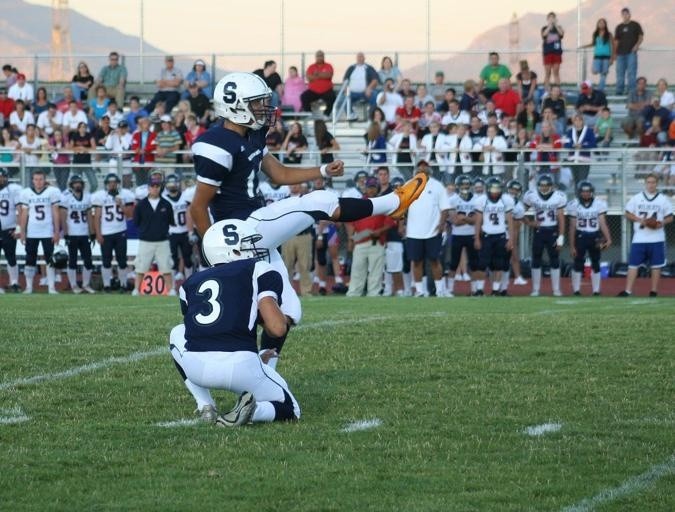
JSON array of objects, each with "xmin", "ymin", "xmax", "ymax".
[{"xmin": 366, "ymin": 177, "xmax": 381, "ymax": 187}]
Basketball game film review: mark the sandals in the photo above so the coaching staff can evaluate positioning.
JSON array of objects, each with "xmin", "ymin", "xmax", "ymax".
[{"xmin": 51, "ymin": 250, "xmax": 67, "ymax": 269}]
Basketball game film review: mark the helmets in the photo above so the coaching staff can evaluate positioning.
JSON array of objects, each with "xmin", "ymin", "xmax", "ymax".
[
  {"xmin": 577, "ymin": 182, "xmax": 595, "ymax": 200},
  {"xmin": 213, "ymin": 74, "xmax": 277, "ymax": 130},
  {"xmin": 486, "ymin": 178, "xmax": 502, "ymax": 199},
  {"xmin": 165, "ymin": 173, "xmax": 180, "ymax": 190},
  {"xmin": 355, "ymin": 171, "xmax": 369, "ymax": 186},
  {"xmin": 455, "ymin": 175, "xmax": 470, "ymax": 192},
  {"xmin": 203, "ymin": 219, "xmax": 271, "ymax": 268},
  {"xmin": 105, "ymin": 174, "xmax": 121, "ymax": 185},
  {"xmin": 148, "ymin": 169, "xmax": 165, "ymax": 185},
  {"xmin": 538, "ymin": 175, "xmax": 553, "ymax": 195},
  {"xmin": 69, "ymin": 175, "xmax": 85, "ymax": 186},
  {"xmin": 473, "ymin": 178, "xmax": 482, "ymax": 186},
  {"xmin": 508, "ymin": 181, "xmax": 523, "ymax": 196}
]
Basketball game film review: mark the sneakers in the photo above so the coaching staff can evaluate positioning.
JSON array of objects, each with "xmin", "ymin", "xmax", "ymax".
[
  {"xmin": 515, "ymin": 277, "xmax": 528, "ymax": 285},
  {"xmin": 23, "ymin": 288, "xmax": 32, "ymax": 294},
  {"xmin": 131, "ymin": 289, "xmax": 139, "ymax": 295},
  {"xmin": 437, "ymin": 291, "xmax": 443, "ymax": 297},
  {"xmin": 463, "ymin": 274, "xmax": 470, "ymax": 281},
  {"xmin": 415, "ymin": 292, "xmax": 424, "ymax": 297},
  {"xmin": 492, "ymin": 290, "xmax": 498, "ymax": 294},
  {"xmin": 48, "ymin": 288, "xmax": 58, "ymax": 293},
  {"xmin": 454, "ymin": 274, "xmax": 462, "ymax": 280},
  {"xmin": 617, "ymin": 291, "xmax": 630, "ymax": 297},
  {"xmin": 649, "ymin": 291, "xmax": 655, "ymax": 297},
  {"xmin": 531, "ymin": 291, "xmax": 539, "ymax": 295},
  {"xmin": 445, "ymin": 291, "xmax": 452, "ymax": 297},
  {"xmin": 103, "ymin": 286, "xmax": 112, "ymax": 293},
  {"xmin": 194, "ymin": 405, "xmax": 216, "ymax": 423},
  {"xmin": 553, "ymin": 291, "xmax": 564, "ymax": 297},
  {"xmin": 215, "ymin": 391, "xmax": 255, "ymax": 429},
  {"xmin": 168, "ymin": 291, "xmax": 178, "ymax": 296},
  {"xmin": 501, "ymin": 291, "xmax": 509, "ymax": 295},
  {"xmin": 120, "ymin": 286, "xmax": 127, "ymax": 292},
  {"xmin": 476, "ymin": 290, "xmax": 484, "ymax": 296},
  {"xmin": 594, "ymin": 292, "xmax": 600, "ymax": 296},
  {"xmin": 574, "ymin": 291, "xmax": 584, "ymax": 297},
  {"xmin": 11, "ymin": 284, "xmax": 22, "ymax": 293},
  {"xmin": 390, "ymin": 173, "xmax": 426, "ymax": 222},
  {"xmin": 83, "ymin": 286, "xmax": 94, "ymax": 293},
  {"xmin": 72, "ymin": 285, "xmax": 81, "ymax": 293}
]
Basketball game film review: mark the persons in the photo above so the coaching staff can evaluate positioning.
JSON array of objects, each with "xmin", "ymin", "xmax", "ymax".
[
  {"xmin": 48, "ymin": 62, "xmax": 94, "ymax": 104},
  {"xmin": 343, "ymin": 53, "xmax": 384, "ymax": 121},
  {"xmin": 562, "ymin": 113, "xmax": 595, "ymax": 190},
  {"xmin": 473, "ymin": 180, "xmax": 486, "ymax": 199},
  {"xmin": 542, "ymin": 86, "xmax": 567, "ymax": 131},
  {"xmin": 147, "ymin": 101, "xmax": 172, "ymax": 133},
  {"xmin": 161, "ymin": 174, "xmax": 195, "ymax": 295},
  {"xmin": 281, "ymin": 67, "xmax": 306, "ymax": 120},
  {"xmin": 309, "ymin": 177, "xmax": 349, "ymax": 295},
  {"xmin": 264, "ymin": 126, "xmax": 283, "ymax": 151},
  {"xmin": 259, "ymin": 177, "xmax": 291, "ymax": 255},
  {"xmin": 459, "ymin": 115, "xmax": 485, "ymax": 178},
  {"xmin": 389, "ymin": 177, "xmax": 412, "ymax": 245},
  {"xmin": 89, "ymin": 86, "xmax": 111, "ymax": 131},
  {"xmin": 0, "ymin": 65, "xmax": 19, "ymax": 127},
  {"xmin": 282, "ymin": 123, "xmax": 307, "ymax": 165},
  {"xmin": 69, "ymin": 123, "xmax": 97, "ymax": 193},
  {"xmin": 314, "ymin": 120, "xmax": 340, "ymax": 187},
  {"xmin": 491, "ymin": 79, "xmax": 523, "ymax": 118},
  {"xmin": 173, "ymin": 112, "xmax": 187, "ymax": 163},
  {"xmin": 17, "ymin": 124, "xmax": 46, "ymax": 186},
  {"xmin": 361, "ymin": 107, "xmax": 385, "ymax": 157},
  {"xmin": 447, "ymin": 174, "xmax": 479, "ymax": 296},
  {"xmin": 460, "ymin": 80, "xmax": 480, "ymax": 114},
  {"xmin": 186, "ymin": 82, "xmax": 213, "ymax": 129},
  {"xmin": 376, "ymin": 78, "xmax": 405, "ymax": 131},
  {"xmin": 578, "ymin": 19, "xmax": 615, "ymax": 90},
  {"xmin": 180, "ymin": 59, "xmax": 211, "ymax": 99},
  {"xmin": 345, "ymin": 179, "xmax": 353, "ymax": 187},
  {"xmin": 300, "ymin": 50, "xmax": 336, "ymax": 117},
  {"xmin": 9, "ymin": 99, "xmax": 35, "ymax": 137},
  {"xmin": 346, "ymin": 177, "xmax": 397, "ymax": 296},
  {"xmin": 47, "ymin": 131, "xmax": 70, "ymax": 192},
  {"xmin": 275, "ymin": 117, "xmax": 288, "ymax": 144},
  {"xmin": 171, "ymin": 100, "xmax": 197, "ymax": 127},
  {"xmin": 529, "ymin": 121, "xmax": 562, "ymax": 185},
  {"xmin": 105, "ymin": 121, "xmax": 133, "ymax": 189},
  {"xmin": 413, "ymin": 83, "xmax": 436, "ymax": 112},
  {"xmin": 419, "ymin": 102, "xmax": 442, "ymax": 139},
  {"xmin": 475, "ymin": 177, "xmax": 515, "ymax": 296},
  {"xmin": 506, "ymin": 181, "xmax": 542, "ymax": 284},
  {"xmin": 32, "ymin": 88, "xmax": 54, "ymax": 124},
  {"xmin": 515, "ymin": 60, "xmax": 539, "ymax": 111},
  {"xmin": 181, "ymin": 184, "xmax": 213, "ymax": 272},
  {"xmin": 620, "ymin": 78, "xmax": 675, "ymax": 179},
  {"xmin": 614, "ymin": 8, "xmax": 644, "ymax": 96},
  {"xmin": 62, "ymin": 101, "xmax": 89, "ymax": 146},
  {"xmin": 127, "ymin": 171, "xmax": 165, "ymax": 278},
  {"xmin": 568, "ymin": 182, "xmax": 612, "ymax": 296},
  {"xmin": 36, "ymin": 104, "xmax": 61, "ymax": 139},
  {"xmin": 520, "ymin": 176, "xmax": 568, "ymax": 296},
  {"xmin": 190, "ymin": 72, "xmax": 427, "ymax": 371},
  {"xmin": 541, "ymin": 12, "xmax": 565, "ymax": 84},
  {"xmin": 477, "ymin": 100, "xmax": 502, "ymax": 125},
  {"xmin": 444, "ymin": 123, "xmax": 457, "ymax": 168},
  {"xmin": 576, "ymin": 83, "xmax": 610, "ymax": 127},
  {"xmin": 55, "ymin": 87, "xmax": 83, "ymax": 113},
  {"xmin": 399, "ymin": 159, "xmax": 455, "ymax": 297},
  {"xmin": 440, "ymin": 101, "xmax": 469, "ymax": 146},
  {"xmin": 122, "ymin": 96, "xmax": 147, "ymax": 135},
  {"xmin": 393, "ymin": 97, "xmax": 424, "ymax": 165},
  {"xmin": 132, "ymin": 178, "xmax": 178, "ymax": 296},
  {"xmin": 483, "ymin": 111, "xmax": 505, "ymax": 138},
  {"xmin": 397, "ymin": 79, "xmax": 418, "ymax": 102},
  {"xmin": 144, "ymin": 56, "xmax": 184, "ymax": 115},
  {"xmin": 517, "ymin": 99, "xmax": 542, "ymax": 130},
  {"xmin": 19, "ymin": 172, "xmax": 66, "ymax": 295},
  {"xmin": 368, "ymin": 122, "xmax": 387, "ymax": 163},
  {"xmin": 497, "ymin": 112, "xmax": 531, "ymax": 149},
  {"xmin": 441, "ymin": 174, "xmax": 457, "ymax": 274},
  {"xmin": 374, "ymin": 167, "xmax": 414, "ymax": 296},
  {"xmin": 542, "ymin": 84, "xmax": 566, "ymax": 110},
  {"xmin": 0, "ymin": 127, "xmax": 21, "ymax": 183},
  {"xmin": 87, "ymin": 52, "xmax": 128, "ymax": 115},
  {"xmin": 477, "ymin": 52, "xmax": 512, "ymax": 105},
  {"xmin": 130, "ymin": 118, "xmax": 157, "ymax": 184},
  {"xmin": 536, "ymin": 106, "xmax": 563, "ymax": 137},
  {"xmin": 436, "ymin": 88, "xmax": 468, "ymax": 118},
  {"xmin": 455, "ymin": 245, "xmax": 472, "ymax": 282},
  {"xmin": 592, "ymin": 108, "xmax": 615, "ymax": 162},
  {"xmin": 59, "ymin": 175, "xmax": 96, "ymax": 293},
  {"xmin": 1, "ymin": 167, "xmax": 25, "ymax": 293},
  {"xmin": 105, "ymin": 101, "xmax": 124, "ymax": 129},
  {"xmin": 151, "ymin": 115, "xmax": 183, "ymax": 179},
  {"xmin": 91, "ymin": 174, "xmax": 136, "ymax": 294},
  {"xmin": 616, "ymin": 173, "xmax": 675, "ymax": 297},
  {"xmin": 341, "ymin": 171, "xmax": 370, "ymax": 286},
  {"xmin": 473, "ymin": 126, "xmax": 506, "ymax": 180},
  {"xmin": 91, "ymin": 116, "xmax": 115, "ymax": 190},
  {"xmin": 377, "ymin": 57, "xmax": 403, "ymax": 87},
  {"xmin": 170, "ymin": 219, "xmax": 302, "ymax": 427},
  {"xmin": 277, "ymin": 183, "xmax": 328, "ymax": 297},
  {"xmin": 8, "ymin": 74, "xmax": 34, "ymax": 111},
  {"xmin": 471, "ymin": 91, "xmax": 490, "ymax": 118},
  {"xmin": 420, "ymin": 123, "xmax": 448, "ymax": 177},
  {"xmin": 252, "ymin": 61, "xmax": 285, "ymax": 112},
  {"xmin": 184, "ymin": 115, "xmax": 206, "ymax": 163}
]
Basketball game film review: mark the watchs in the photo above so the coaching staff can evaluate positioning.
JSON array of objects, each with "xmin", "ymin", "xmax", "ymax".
[{"xmin": 317, "ymin": 236, "xmax": 323, "ymax": 241}]
